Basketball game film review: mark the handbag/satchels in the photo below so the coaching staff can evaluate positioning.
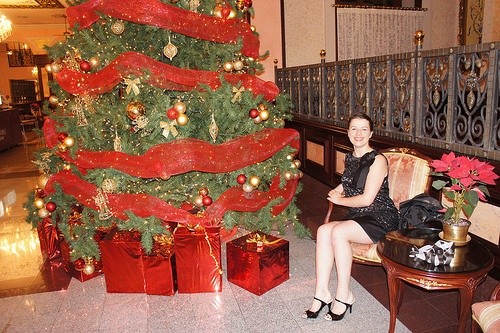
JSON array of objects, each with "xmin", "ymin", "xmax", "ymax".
[{"xmin": 400, "ymin": 193, "xmax": 443, "ymax": 238}]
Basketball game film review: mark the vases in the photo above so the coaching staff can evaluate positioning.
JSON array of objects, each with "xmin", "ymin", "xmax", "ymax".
[{"xmin": 443, "ymin": 217, "xmax": 471, "ymax": 242}]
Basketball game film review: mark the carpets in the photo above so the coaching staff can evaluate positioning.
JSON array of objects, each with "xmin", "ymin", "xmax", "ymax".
[{"xmin": 0, "ymin": 220, "xmax": 412, "ymax": 333}]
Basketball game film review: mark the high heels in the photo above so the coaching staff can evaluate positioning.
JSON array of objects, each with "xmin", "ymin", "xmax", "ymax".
[
  {"xmin": 324, "ymin": 292, "xmax": 355, "ymax": 322},
  {"xmin": 301, "ymin": 291, "xmax": 334, "ymax": 319}
]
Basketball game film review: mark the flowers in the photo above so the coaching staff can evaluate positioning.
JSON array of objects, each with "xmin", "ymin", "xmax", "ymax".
[{"xmin": 426, "ymin": 152, "xmax": 500, "ymax": 225}]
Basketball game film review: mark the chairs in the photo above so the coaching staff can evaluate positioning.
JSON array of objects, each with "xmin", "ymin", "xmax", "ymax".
[
  {"xmin": 320, "ymin": 147, "xmax": 434, "ymax": 315},
  {"xmin": 472, "ymin": 284, "xmax": 500, "ymax": 333}
]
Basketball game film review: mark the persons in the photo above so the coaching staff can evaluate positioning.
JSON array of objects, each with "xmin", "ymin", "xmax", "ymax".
[
  {"xmin": 301, "ymin": 112, "xmax": 399, "ymax": 321},
  {"xmin": 3, "ymin": 95, "xmax": 13, "ymax": 108}
]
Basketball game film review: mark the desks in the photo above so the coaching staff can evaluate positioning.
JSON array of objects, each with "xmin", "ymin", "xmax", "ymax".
[
  {"xmin": 0, "ymin": 106, "xmax": 22, "ymax": 152},
  {"xmin": 378, "ymin": 226, "xmax": 495, "ymax": 333}
]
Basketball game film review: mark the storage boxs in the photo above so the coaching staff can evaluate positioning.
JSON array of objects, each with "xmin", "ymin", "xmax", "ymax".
[
  {"xmin": 227, "ymin": 231, "xmax": 290, "ymax": 296},
  {"xmin": 99, "ymin": 224, "xmax": 177, "ymax": 296},
  {"xmin": 58, "ymin": 234, "xmax": 102, "ymax": 283},
  {"xmin": 172, "ymin": 227, "xmax": 222, "ymax": 292}
]
